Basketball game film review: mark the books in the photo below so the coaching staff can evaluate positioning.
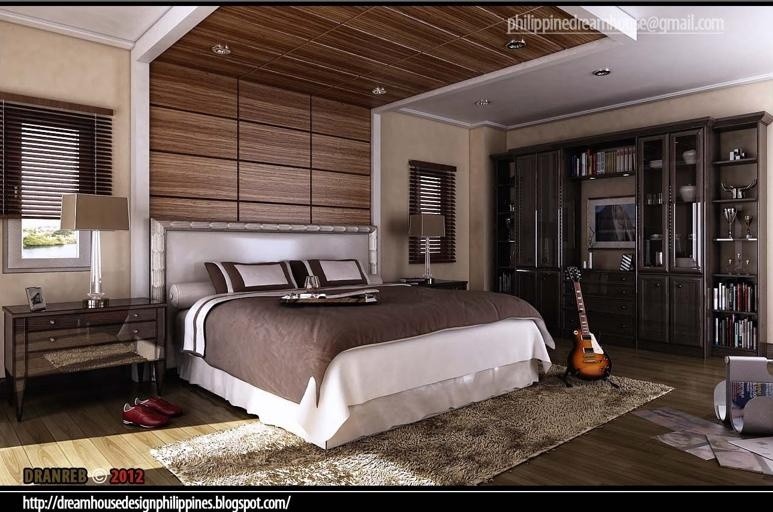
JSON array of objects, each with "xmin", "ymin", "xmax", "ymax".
[
  {"xmin": 571, "ymin": 144, "xmax": 635, "ymax": 176},
  {"xmin": 713, "ymin": 281, "xmax": 757, "ymax": 351},
  {"xmin": 400, "ymin": 277, "xmax": 425, "ymax": 283}
]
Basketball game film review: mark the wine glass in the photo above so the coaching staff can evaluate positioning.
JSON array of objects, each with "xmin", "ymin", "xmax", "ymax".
[{"xmin": 303, "ymin": 276, "xmax": 320, "ymax": 300}]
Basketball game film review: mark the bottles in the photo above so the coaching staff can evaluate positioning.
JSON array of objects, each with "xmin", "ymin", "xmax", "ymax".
[{"xmin": 583, "ymin": 251, "xmax": 593, "ymax": 270}]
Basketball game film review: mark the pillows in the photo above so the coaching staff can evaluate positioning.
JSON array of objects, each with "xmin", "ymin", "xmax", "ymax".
[{"xmin": 169, "ymin": 258, "xmax": 384, "ymax": 309}]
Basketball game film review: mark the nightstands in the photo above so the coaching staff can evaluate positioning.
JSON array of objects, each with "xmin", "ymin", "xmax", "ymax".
[
  {"xmin": 2, "ymin": 298, "xmax": 167, "ymax": 422},
  {"xmin": 385, "ymin": 279, "xmax": 468, "ymax": 291}
]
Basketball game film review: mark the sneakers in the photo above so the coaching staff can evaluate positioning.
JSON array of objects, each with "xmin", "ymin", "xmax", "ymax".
[
  {"xmin": 134, "ymin": 395, "xmax": 182, "ymax": 418},
  {"xmin": 122, "ymin": 402, "xmax": 167, "ymax": 429}
]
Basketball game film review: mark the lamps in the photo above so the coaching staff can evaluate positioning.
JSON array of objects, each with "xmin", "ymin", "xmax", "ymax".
[
  {"xmin": 408, "ymin": 215, "xmax": 445, "ymax": 282},
  {"xmin": 60, "ymin": 193, "xmax": 129, "ymax": 306}
]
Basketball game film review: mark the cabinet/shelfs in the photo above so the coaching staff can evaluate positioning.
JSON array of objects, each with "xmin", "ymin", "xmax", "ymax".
[{"xmin": 488, "ymin": 110, "xmax": 773, "ymax": 361}]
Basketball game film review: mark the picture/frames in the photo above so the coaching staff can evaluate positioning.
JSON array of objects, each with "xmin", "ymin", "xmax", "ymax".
[{"xmin": 25, "ymin": 287, "xmax": 46, "ymax": 311}]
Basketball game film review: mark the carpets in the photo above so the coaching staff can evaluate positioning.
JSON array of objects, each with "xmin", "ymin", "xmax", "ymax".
[{"xmin": 150, "ymin": 362, "xmax": 675, "ymax": 486}]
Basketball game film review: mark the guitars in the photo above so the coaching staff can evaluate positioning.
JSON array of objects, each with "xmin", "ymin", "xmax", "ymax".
[{"xmin": 564, "ymin": 267, "xmax": 612, "ymax": 381}]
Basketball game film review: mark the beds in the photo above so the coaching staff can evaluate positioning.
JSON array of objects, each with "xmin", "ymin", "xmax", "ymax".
[{"xmin": 150, "ymin": 216, "xmax": 556, "ymax": 451}]
